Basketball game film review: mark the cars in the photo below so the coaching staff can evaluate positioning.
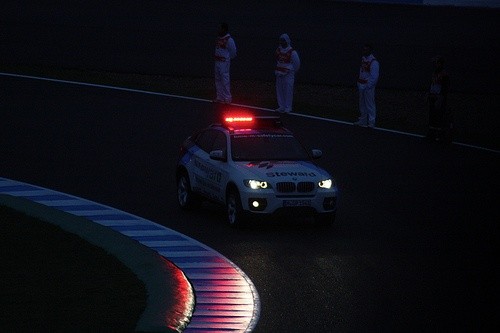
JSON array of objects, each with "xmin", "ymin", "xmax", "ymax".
[{"xmin": 177, "ymin": 114, "xmax": 337, "ymax": 228}]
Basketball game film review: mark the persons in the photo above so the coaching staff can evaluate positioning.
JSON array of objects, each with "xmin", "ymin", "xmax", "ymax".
[
  {"xmin": 357, "ymin": 47, "xmax": 380, "ymax": 128},
  {"xmin": 273, "ymin": 33, "xmax": 300, "ymax": 113},
  {"xmin": 213, "ymin": 22, "xmax": 237, "ymax": 104}
]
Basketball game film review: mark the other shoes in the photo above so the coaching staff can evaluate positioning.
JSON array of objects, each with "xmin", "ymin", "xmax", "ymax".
[
  {"xmin": 366, "ymin": 124, "xmax": 375, "ymax": 128},
  {"xmin": 274, "ymin": 108, "xmax": 290, "ymax": 114},
  {"xmin": 354, "ymin": 121, "xmax": 368, "ymax": 126},
  {"xmin": 213, "ymin": 98, "xmax": 230, "ymax": 105}
]
